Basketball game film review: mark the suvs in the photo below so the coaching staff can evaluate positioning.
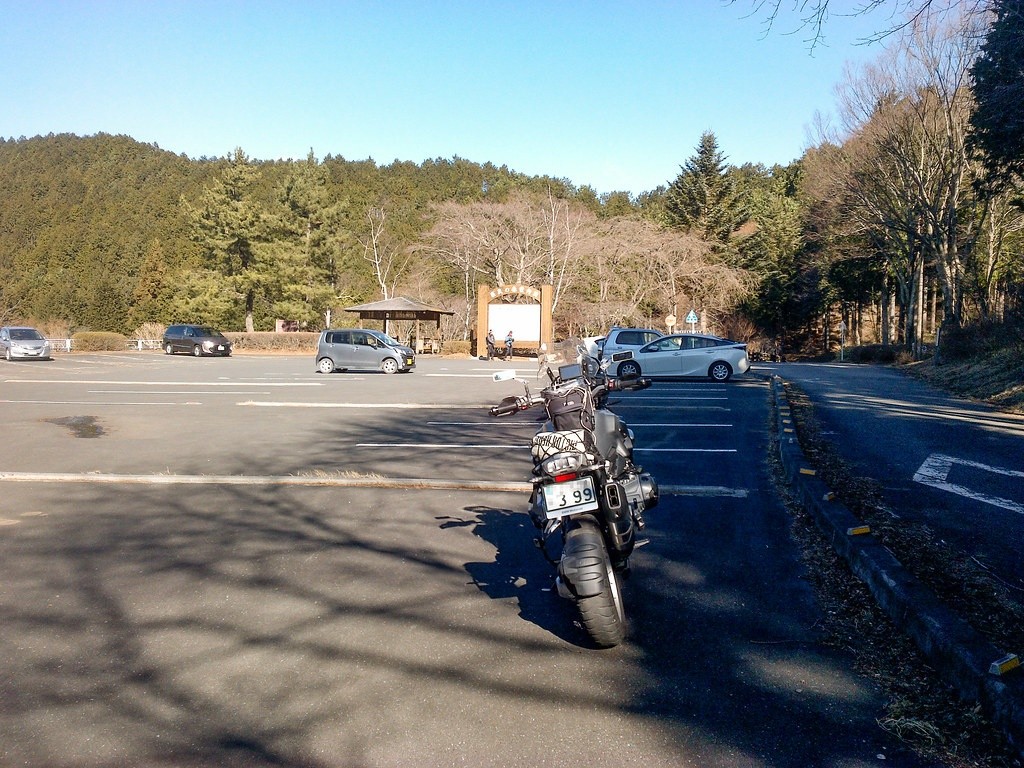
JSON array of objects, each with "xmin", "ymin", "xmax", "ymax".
[{"xmin": 597, "ymin": 327, "xmax": 680, "ymax": 361}]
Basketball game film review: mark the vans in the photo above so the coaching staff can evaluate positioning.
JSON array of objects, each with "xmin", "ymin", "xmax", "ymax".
[{"xmin": 161, "ymin": 325, "xmax": 232, "ymax": 356}]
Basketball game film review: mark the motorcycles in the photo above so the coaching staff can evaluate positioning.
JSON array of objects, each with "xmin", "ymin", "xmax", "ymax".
[{"xmin": 486, "ymin": 335, "xmax": 659, "ymax": 647}]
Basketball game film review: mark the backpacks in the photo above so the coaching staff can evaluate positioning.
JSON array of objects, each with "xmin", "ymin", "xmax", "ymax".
[{"xmin": 541, "ymin": 386, "xmax": 596, "ymax": 431}]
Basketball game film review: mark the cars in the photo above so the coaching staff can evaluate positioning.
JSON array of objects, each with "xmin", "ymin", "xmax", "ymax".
[
  {"xmin": 604, "ymin": 330, "xmax": 679, "ymax": 359},
  {"xmin": 607, "ymin": 333, "xmax": 750, "ymax": 380},
  {"xmin": 0, "ymin": 326, "xmax": 51, "ymax": 361},
  {"xmin": 316, "ymin": 328, "xmax": 417, "ymax": 375}
]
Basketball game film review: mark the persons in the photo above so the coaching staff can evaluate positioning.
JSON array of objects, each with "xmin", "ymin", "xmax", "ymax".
[
  {"xmin": 486, "ymin": 330, "xmax": 496, "ymax": 360},
  {"xmin": 504, "ymin": 332, "xmax": 514, "ymax": 362}
]
